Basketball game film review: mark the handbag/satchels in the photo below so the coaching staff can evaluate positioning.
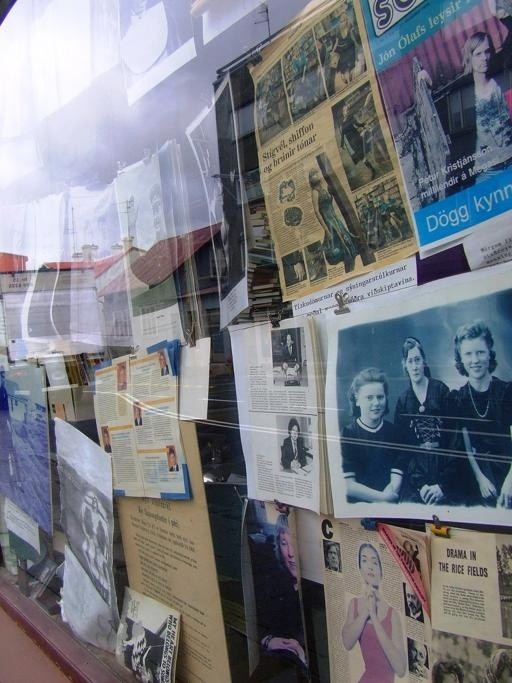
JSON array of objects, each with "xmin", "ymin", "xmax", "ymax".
[
  {"xmin": 390, "ymin": 211, "xmax": 404, "ymax": 227},
  {"xmin": 355, "ymin": 53, "xmax": 365, "ymax": 76},
  {"xmin": 325, "ymin": 247, "xmax": 343, "ymax": 265},
  {"xmin": 350, "ymin": 152, "xmax": 360, "ymax": 164}
]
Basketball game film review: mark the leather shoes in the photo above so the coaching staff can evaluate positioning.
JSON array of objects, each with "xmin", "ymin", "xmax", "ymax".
[{"xmin": 284, "ymin": 380, "xmax": 301, "ymax": 386}]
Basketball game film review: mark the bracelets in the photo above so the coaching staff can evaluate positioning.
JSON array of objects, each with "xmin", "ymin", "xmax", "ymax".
[{"xmin": 261, "ymin": 632, "xmax": 276, "ymax": 649}]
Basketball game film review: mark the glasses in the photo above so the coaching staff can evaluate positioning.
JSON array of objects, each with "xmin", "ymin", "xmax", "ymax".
[{"xmin": 340, "ymin": 20, "xmax": 345, "ymax": 23}]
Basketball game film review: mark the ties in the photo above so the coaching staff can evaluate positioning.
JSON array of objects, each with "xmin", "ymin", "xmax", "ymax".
[{"xmin": 294, "ymin": 442, "xmax": 297, "ymax": 455}]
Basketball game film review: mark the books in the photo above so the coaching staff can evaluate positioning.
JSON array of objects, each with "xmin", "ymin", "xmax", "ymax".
[{"xmin": 114, "ymin": 585, "xmax": 183, "ymax": 683}]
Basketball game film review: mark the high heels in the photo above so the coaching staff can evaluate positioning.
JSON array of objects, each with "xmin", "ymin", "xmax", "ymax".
[{"xmin": 341, "ymin": 73, "xmax": 353, "ymax": 84}]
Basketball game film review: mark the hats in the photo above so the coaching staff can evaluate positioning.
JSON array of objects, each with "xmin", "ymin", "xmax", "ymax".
[
  {"xmin": 317, "ymin": 30, "xmax": 330, "ymax": 40},
  {"xmin": 335, "ymin": 10, "xmax": 347, "ymax": 17}
]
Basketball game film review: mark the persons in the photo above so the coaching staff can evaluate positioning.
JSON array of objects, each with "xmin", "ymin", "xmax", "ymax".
[
  {"xmin": 399, "ymin": 584, "xmax": 425, "ymax": 624},
  {"xmin": 481, "ymin": 651, "xmax": 511, "ymax": 683},
  {"xmin": 429, "ymin": 660, "xmax": 464, "ymax": 683},
  {"xmin": 289, "ymin": 45, "xmax": 314, "ymax": 90},
  {"xmin": 164, "ymin": 445, "xmax": 180, "ymax": 472},
  {"xmin": 132, "ymin": 400, "xmax": 143, "ymax": 427},
  {"xmin": 454, "ymin": 0, "xmax": 512, "ymax": 181},
  {"xmin": 403, "ymin": 637, "xmax": 430, "ymax": 678},
  {"xmin": 158, "ymin": 349, "xmax": 171, "ymax": 376},
  {"xmin": 306, "ymin": 165, "xmax": 361, "ymax": 273},
  {"xmin": 326, "ymin": 7, "xmax": 363, "ymax": 86},
  {"xmin": 276, "ymin": 329, "xmax": 303, "ymax": 388},
  {"xmin": 341, "ymin": 541, "xmax": 408, "ymax": 682},
  {"xmin": 102, "ymin": 425, "xmax": 112, "ymax": 453},
  {"xmin": 334, "ymin": 102, "xmax": 379, "ymax": 183},
  {"xmin": 284, "ymin": 254, "xmax": 308, "ymax": 282},
  {"xmin": 320, "ymin": 540, "xmax": 339, "ymax": 574},
  {"xmin": 275, "ymin": 418, "xmax": 311, "ymax": 476},
  {"xmin": 240, "ymin": 511, "xmax": 311, "ymax": 682},
  {"xmin": 393, "ymin": 335, "xmax": 461, "ymax": 505},
  {"xmin": 451, "ymin": 318, "xmax": 511, "ymax": 510},
  {"xmin": 338, "ymin": 366, "xmax": 409, "ymax": 505},
  {"xmin": 116, "ymin": 362, "xmax": 128, "ymax": 391}
]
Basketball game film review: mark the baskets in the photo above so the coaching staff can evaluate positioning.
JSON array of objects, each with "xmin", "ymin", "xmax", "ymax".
[{"xmin": 329, "ymin": 52, "xmax": 340, "ymax": 68}]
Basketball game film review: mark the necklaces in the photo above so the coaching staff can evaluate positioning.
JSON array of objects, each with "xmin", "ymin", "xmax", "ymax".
[{"xmin": 464, "ymin": 376, "xmax": 493, "ymax": 419}]
